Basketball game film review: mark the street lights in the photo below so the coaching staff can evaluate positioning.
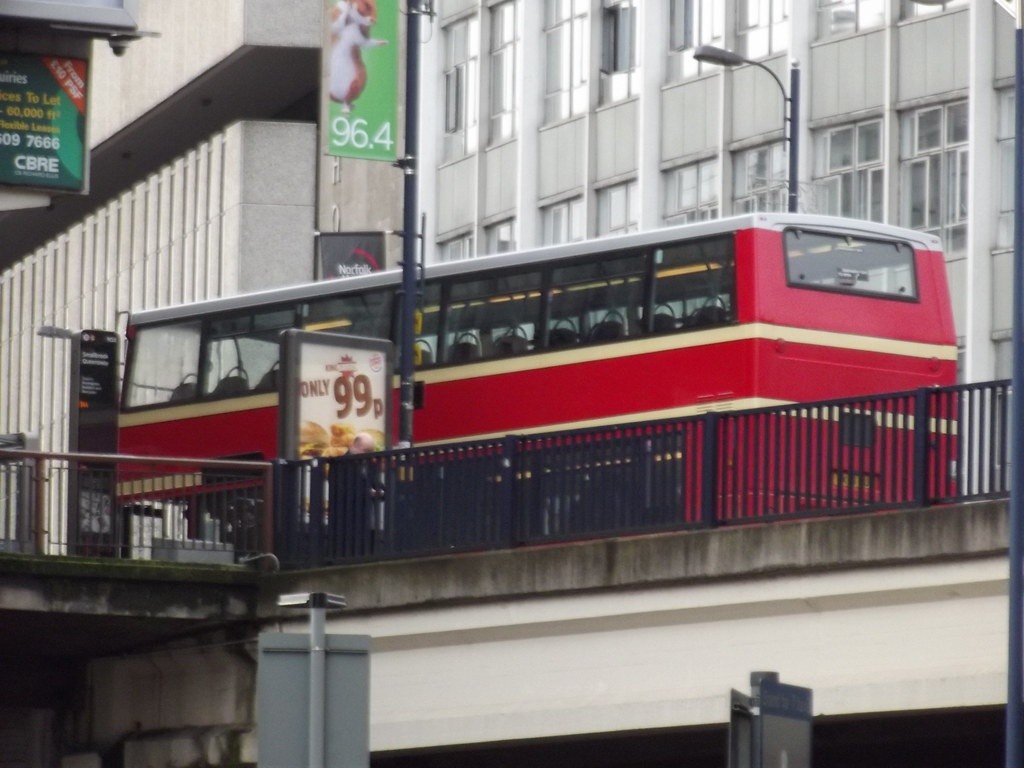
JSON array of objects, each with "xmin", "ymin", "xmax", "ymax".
[{"xmin": 694, "ymin": 44, "xmax": 797, "ymax": 211}]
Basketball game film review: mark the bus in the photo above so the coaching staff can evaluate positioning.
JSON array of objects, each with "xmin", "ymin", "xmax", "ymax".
[{"xmin": 109, "ymin": 211, "xmax": 961, "ymax": 569}]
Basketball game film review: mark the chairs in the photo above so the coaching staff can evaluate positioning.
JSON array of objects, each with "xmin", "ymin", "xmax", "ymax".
[
  {"xmin": 414, "ymin": 336, "xmax": 435, "ymax": 364},
  {"xmin": 697, "ymin": 292, "xmax": 729, "ymax": 327},
  {"xmin": 446, "ymin": 331, "xmax": 480, "ymax": 361},
  {"xmin": 214, "ymin": 366, "xmax": 250, "ymax": 395},
  {"xmin": 548, "ymin": 316, "xmax": 578, "ymax": 350},
  {"xmin": 652, "ymin": 301, "xmax": 676, "ymax": 337},
  {"xmin": 169, "ymin": 373, "xmax": 196, "ymax": 400},
  {"xmin": 592, "ymin": 309, "xmax": 627, "ymax": 341},
  {"xmin": 494, "ymin": 322, "xmax": 529, "ymax": 357},
  {"xmin": 259, "ymin": 359, "xmax": 280, "ymax": 393}
]
